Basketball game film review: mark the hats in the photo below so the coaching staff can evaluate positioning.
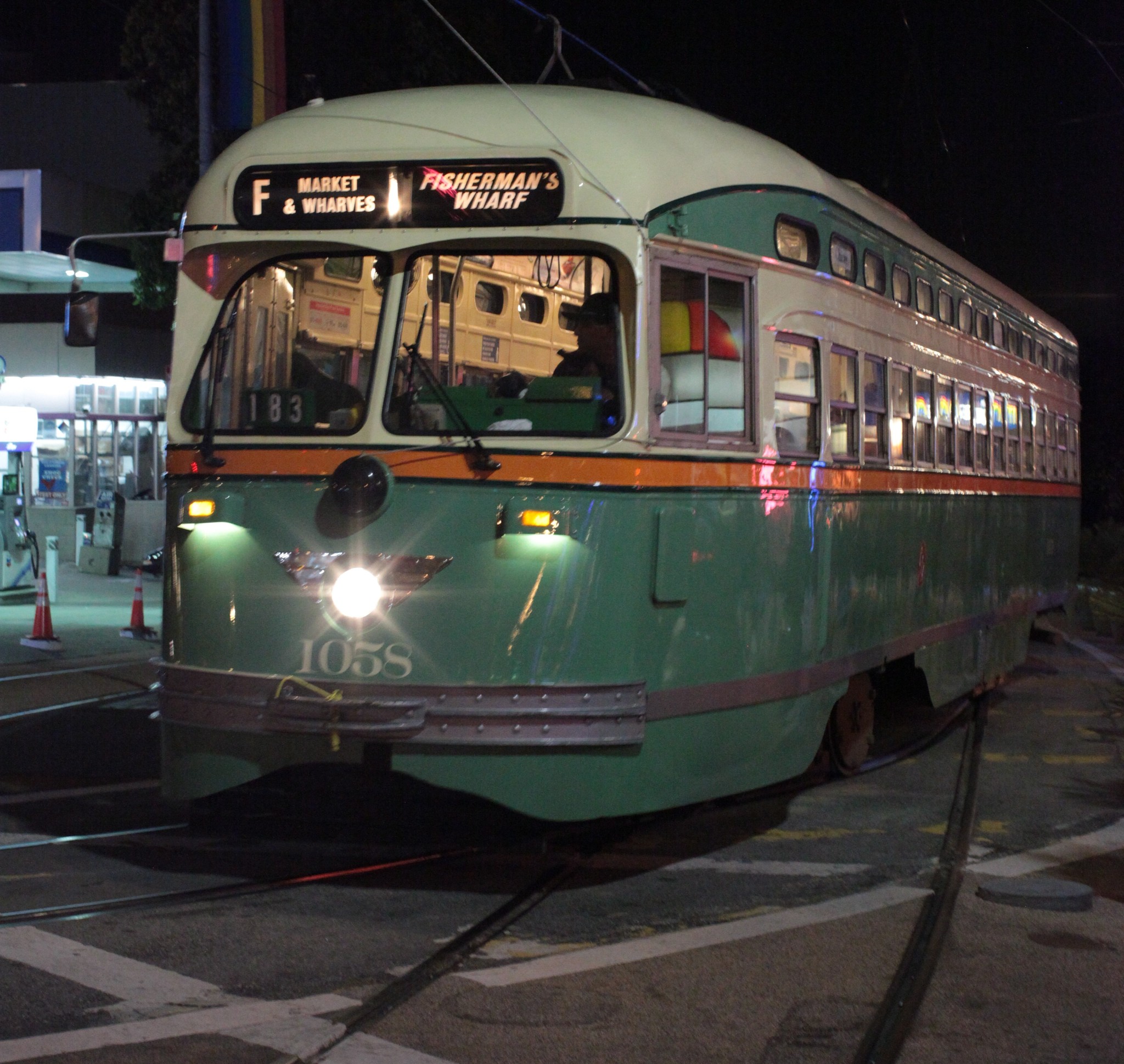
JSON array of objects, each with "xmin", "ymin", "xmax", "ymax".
[{"xmin": 561, "ymin": 292, "xmax": 621, "ymax": 323}]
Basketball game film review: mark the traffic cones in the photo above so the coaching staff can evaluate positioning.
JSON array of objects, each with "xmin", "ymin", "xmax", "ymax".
[
  {"xmin": 24, "ymin": 572, "xmax": 61, "ymax": 641},
  {"xmin": 119, "ymin": 568, "xmax": 152, "ymax": 631}
]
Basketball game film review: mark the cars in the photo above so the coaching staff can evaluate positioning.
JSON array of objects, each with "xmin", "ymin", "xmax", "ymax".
[{"xmin": 140, "ymin": 546, "xmax": 164, "ymax": 577}]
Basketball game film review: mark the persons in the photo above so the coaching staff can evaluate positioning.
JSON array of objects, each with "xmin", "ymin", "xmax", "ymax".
[
  {"xmin": 551, "ymin": 292, "xmax": 619, "ymax": 428},
  {"xmin": 495, "ymin": 368, "xmax": 528, "ymax": 398}
]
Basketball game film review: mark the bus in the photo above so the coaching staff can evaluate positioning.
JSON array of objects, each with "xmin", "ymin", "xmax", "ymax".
[{"xmin": 63, "ymin": 82, "xmax": 1083, "ymax": 826}]
